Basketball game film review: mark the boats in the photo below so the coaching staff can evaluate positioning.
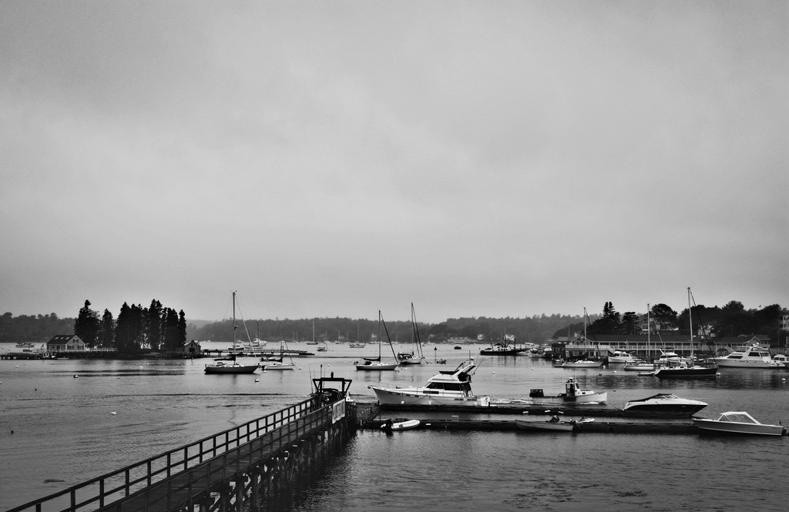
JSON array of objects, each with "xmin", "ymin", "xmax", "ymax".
[
  {"xmin": 369, "ymin": 357, "xmax": 491, "ymax": 407},
  {"xmin": 489, "ymin": 378, "xmax": 786, "ymax": 437},
  {"xmin": 379, "ymin": 417, "xmax": 420, "ymax": 429}
]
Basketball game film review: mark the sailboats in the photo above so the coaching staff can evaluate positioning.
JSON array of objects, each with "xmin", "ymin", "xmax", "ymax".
[
  {"xmin": 356, "ymin": 303, "xmax": 446, "ymax": 370},
  {"xmin": 204, "ymin": 290, "xmax": 296, "ymax": 372}
]
